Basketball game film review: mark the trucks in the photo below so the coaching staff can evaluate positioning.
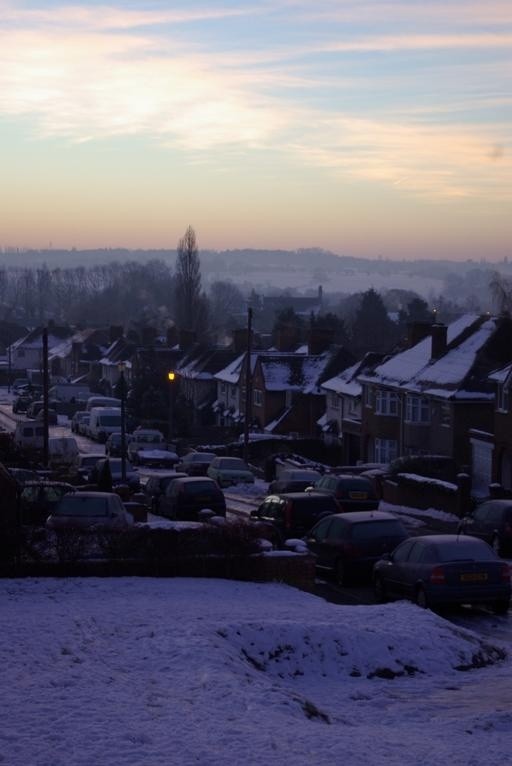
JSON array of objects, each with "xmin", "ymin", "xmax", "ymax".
[{"xmin": 126, "ymin": 428, "xmax": 177, "ymax": 468}]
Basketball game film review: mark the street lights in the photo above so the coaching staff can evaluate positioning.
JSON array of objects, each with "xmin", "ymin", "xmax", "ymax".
[
  {"xmin": 117, "ymin": 359, "xmax": 129, "ymax": 482},
  {"xmin": 432, "ymin": 306, "xmax": 439, "ymax": 321},
  {"xmin": 168, "ymin": 370, "xmax": 177, "ymax": 440}
]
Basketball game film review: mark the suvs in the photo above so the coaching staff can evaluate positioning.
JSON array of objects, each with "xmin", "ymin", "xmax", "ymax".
[
  {"xmin": 305, "ymin": 471, "xmax": 380, "ymax": 511},
  {"xmin": 157, "ymin": 475, "xmax": 226, "ymax": 519},
  {"xmin": 362, "ymin": 455, "xmax": 471, "ymax": 502},
  {"xmin": 250, "ymin": 489, "xmax": 342, "ymax": 540},
  {"xmin": 143, "ymin": 473, "xmax": 190, "ymax": 512}
]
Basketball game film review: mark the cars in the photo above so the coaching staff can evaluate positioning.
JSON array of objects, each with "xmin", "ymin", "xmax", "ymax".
[
  {"xmin": 268, "ymin": 468, "xmax": 321, "ymax": 495},
  {"xmin": 206, "ymin": 456, "xmax": 255, "ymax": 488},
  {"xmin": 0, "ymin": 381, "xmax": 147, "ymax": 529},
  {"xmin": 378, "ymin": 533, "xmax": 512, "ymax": 616},
  {"xmin": 459, "ymin": 497, "xmax": 512, "ymax": 560},
  {"xmin": 175, "ymin": 450, "xmax": 216, "ymax": 473},
  {"xmin": 301, "ymin": 510, "xmax": 406, "ymax": 591}
]
